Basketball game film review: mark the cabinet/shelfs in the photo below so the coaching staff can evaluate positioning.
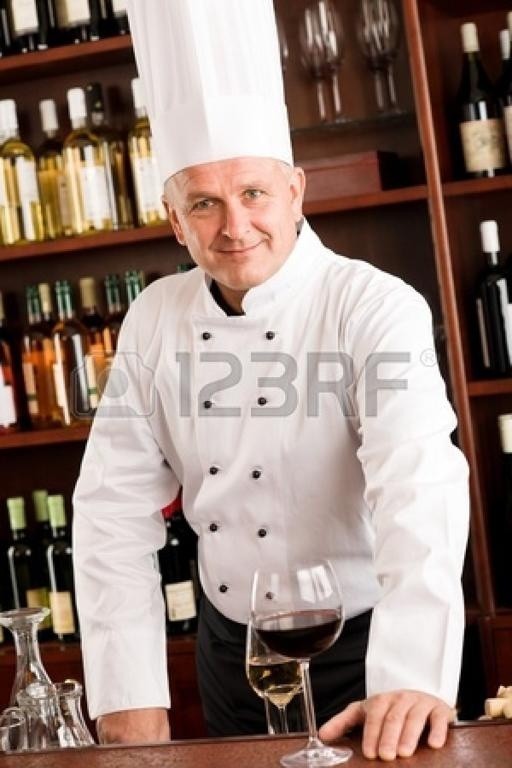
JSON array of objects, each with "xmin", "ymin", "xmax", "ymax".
[{"xmin": 0, "ymin": 0, "xmax": 512, "ymax": 741}]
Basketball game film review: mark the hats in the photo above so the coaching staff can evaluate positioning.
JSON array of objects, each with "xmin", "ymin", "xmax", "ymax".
[{"xmin": 120, "ymin": 0, "xmax": 293, "ymax": 188}]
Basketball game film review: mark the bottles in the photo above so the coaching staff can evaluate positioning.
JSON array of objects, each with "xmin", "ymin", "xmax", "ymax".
[
  {"xmin": 0, "ymin": 1, "xmax": 167, "ymax": 431},
  {"xmin": 474, "ymin": 216, "xmax": 509, "ymax": 378},
  {"xmin": 5, "ymin": 484, "xmax": 201, "ymax": 643},
  {"xmin": 456, "ymin": 19, "xmax": 509, "ymax": 179},
  {"xmin": 0, "ymin": 607, "xmax": 96, "ymax": 754}
]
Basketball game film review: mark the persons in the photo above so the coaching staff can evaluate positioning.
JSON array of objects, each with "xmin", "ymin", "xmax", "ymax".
[{"xmin": 68, "ymin": 155, "xmax": 476, "ymax": 763}]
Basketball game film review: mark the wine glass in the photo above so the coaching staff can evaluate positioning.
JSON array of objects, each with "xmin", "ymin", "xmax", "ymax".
[
  {"xmin": 249, "ymin": 555, "xmax": 354, "ymax": 768},
  {"xmin": 299, "ymin": 0, "xmax": 402, "ymax": 126},
  {"xmin": 244, "ymin": 617, "xmax": 304, "ymax": 736}
]
